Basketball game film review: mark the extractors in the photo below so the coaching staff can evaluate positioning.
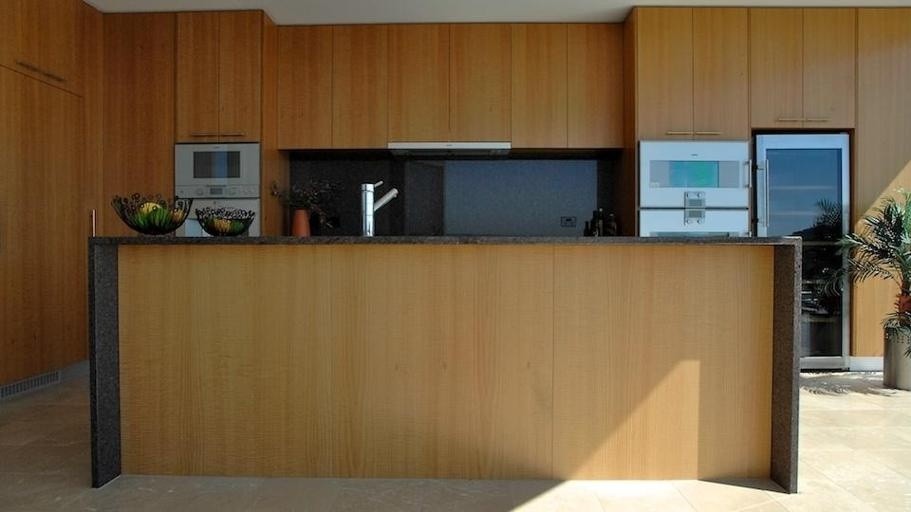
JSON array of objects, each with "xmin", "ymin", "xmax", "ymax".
[{"xmin": 385, "ymin": 140, "xmax": 513, "ymax": 165}]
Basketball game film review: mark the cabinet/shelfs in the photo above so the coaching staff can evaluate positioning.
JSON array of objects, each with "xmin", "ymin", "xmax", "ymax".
[
  {"xmin": 175, "ymin": 10, "xmax": 278, "ymax": 145},
  {"xmin": 623, "ymin": 6, "xmax": 751, "ymax": 140},
  {"xmin": 754, "ymin": 133, "xmax": 851, "ymax": 371},
  {"xmin": 751, "ymin": 7, "xmax": 856, "ymax": 130},
  {"xmin": 83, "ymin": 0, "xmax": 101, "ymax": 362},
  {"xmin": 0, "ymin": 0, "xmax": 84, "ymax": 98}
]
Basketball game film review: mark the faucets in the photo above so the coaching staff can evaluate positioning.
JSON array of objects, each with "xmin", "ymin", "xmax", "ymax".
[{"xmin": 359, "ymin": 180, "xmax": 399, "ymax": 238}]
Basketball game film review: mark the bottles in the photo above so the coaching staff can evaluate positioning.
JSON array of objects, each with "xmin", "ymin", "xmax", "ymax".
[{"xmin": 590, "ymin": 208, "xmax": 620, "ymax": 236}]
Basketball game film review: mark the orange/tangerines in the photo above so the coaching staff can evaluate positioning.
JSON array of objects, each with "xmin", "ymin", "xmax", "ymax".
[
  {"xmin": 205, "ymin": 214, "xmax": 243, "ymax": 233},
  {"xmin": 131, "ymin": 202, "xmax": 184, "ymax": 225}
]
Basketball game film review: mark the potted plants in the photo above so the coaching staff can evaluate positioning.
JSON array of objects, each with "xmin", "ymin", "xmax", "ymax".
[
  {"xmin": 272, "ymin": 177, "xmax": 335, "ymax": 236},
  {"xmin": 825, "ymin": 193, "xmax": 911, "ymax": 357}
]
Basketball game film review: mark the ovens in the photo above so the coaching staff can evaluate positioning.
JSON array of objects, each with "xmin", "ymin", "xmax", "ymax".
[{"xmin": 638, "ymin": 139, "xmax": 752, "ymax": 239}]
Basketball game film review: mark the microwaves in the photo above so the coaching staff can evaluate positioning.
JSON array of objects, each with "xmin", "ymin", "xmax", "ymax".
[{"xmin": 174, "ymin": 139, "xmax": 262, "ymax": 200}]
